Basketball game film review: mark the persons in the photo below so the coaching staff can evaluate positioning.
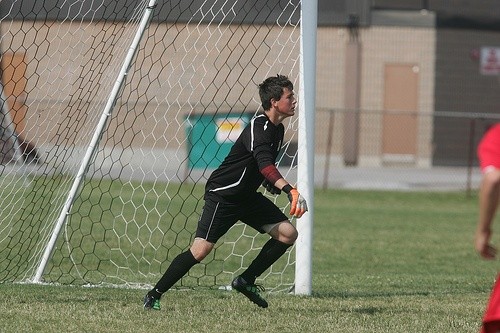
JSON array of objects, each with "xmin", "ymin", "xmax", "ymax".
[
  {"xmin": 477, "ymin": 122, "xmax": 500, "ymax": 333},
  {"xmin": 143, "ymin": 73, "xmax": 307, "ymax": 312}
]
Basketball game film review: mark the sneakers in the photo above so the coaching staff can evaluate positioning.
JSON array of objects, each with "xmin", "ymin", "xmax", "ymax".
[
  {"xmin": 143, "ymin": 291, "xmax": 161, "ymax": 312},
  {"xmin": 231, "ymin": 275, "xmax": 269, "ymax": 308}
]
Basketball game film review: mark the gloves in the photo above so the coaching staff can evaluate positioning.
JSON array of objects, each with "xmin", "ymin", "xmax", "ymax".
[
  {"xmin": 287, "ymin": 188, "xmax": 308, "ymax": 219},
  {"xmin": 263, "ymin": 182, "xmax": 282, "ymax": 195}
]
